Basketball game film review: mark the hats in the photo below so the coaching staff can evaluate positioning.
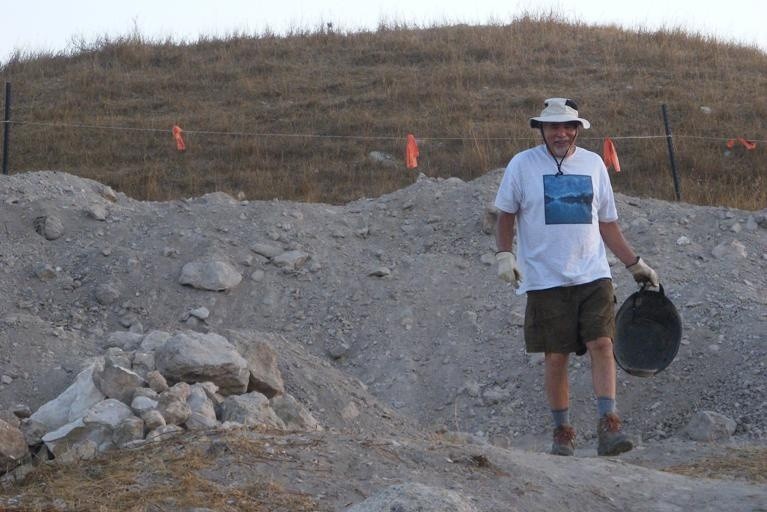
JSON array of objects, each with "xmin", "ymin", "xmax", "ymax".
[{"xmin": 529, "ymin": 97, "xmax": 592, "ymax": 130}]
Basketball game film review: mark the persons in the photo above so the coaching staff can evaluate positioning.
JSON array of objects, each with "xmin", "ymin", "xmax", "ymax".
[{"xmin": 494, "ymin": 98, "xmax": 659, "ymax": 456}]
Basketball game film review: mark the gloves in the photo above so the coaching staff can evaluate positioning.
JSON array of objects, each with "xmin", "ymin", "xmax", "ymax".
[
  {"xmin": 494, "ymin": 251, "xmax": 523, "ymax": 291},
  {"xmin": 626, "ymin": 254, "xmax": 660, "ymax": 289}
]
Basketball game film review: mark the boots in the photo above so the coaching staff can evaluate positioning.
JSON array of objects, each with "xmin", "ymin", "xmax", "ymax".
[
  {"xmin": 552, "ymin": 425, "xmax": 577, "ymax": 456},
  {"xmin": 597, "ymin": 412, "xmax": 633, "ymax": 455}
]
